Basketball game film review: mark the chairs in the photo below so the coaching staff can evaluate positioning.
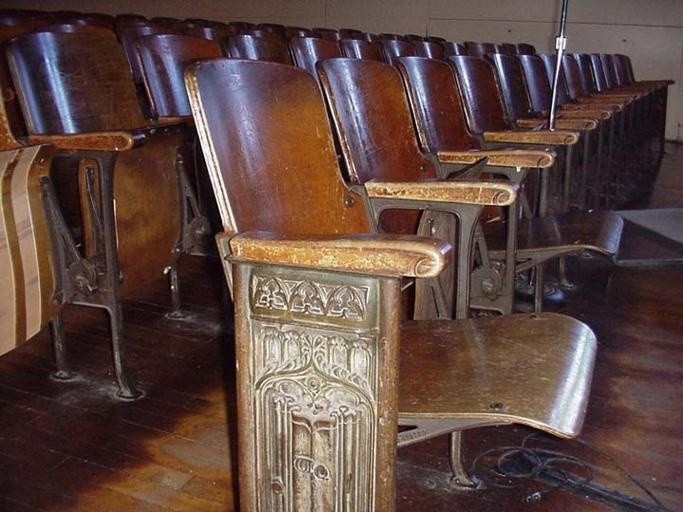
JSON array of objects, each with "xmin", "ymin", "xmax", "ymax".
[{"xmin": 0, "ymin": 9, "xmax": 674, "ymax": 507}]
